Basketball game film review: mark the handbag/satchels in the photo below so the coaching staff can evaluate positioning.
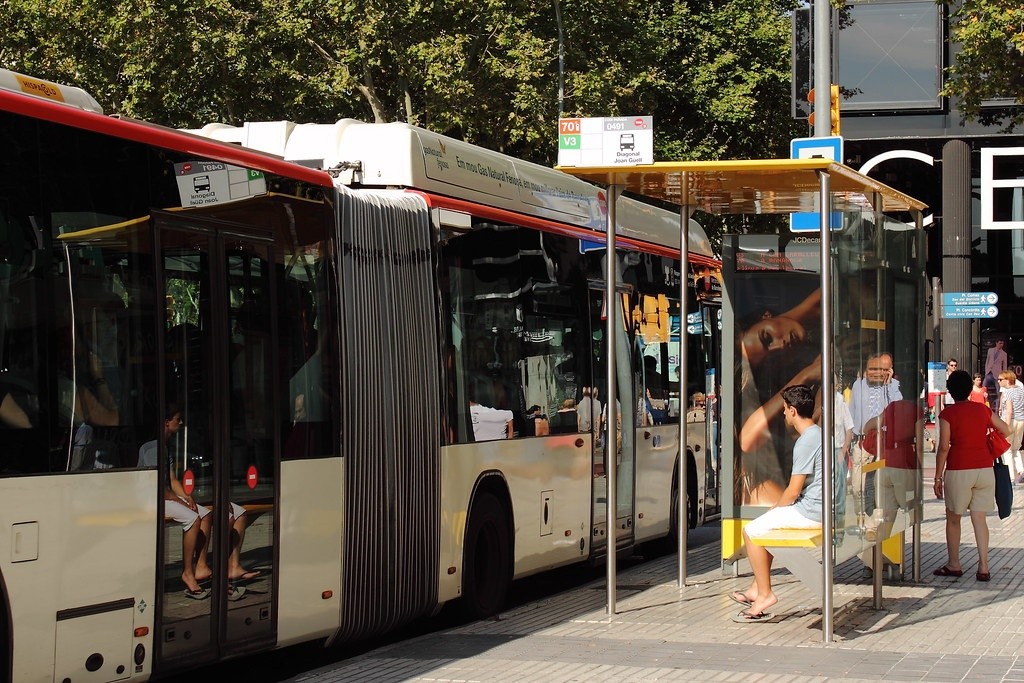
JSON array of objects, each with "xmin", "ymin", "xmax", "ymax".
[
  {"xmin": 993, "ymin": 457, "xmax": 1013, "ymax": 520},
  {"xmin": 984, "ymin": 405, "xmax": 1011, "ymax": 459}
]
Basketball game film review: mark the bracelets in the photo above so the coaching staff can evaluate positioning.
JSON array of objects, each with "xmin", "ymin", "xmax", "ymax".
[
  {"xmin": 95, "ymin": 377, "xmax": 106, "ymax": 385},
  {"xmin": 935, "ymin": 477, "xmax": 943, "ymax": 482}
]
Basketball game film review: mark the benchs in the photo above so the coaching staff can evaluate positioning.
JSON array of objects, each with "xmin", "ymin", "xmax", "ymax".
[{"xmin": 751, "ymin": 528, "xmax": 823, "ymax": 594}]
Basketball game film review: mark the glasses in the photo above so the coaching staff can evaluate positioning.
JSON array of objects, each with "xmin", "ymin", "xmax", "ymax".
[
  {"xmin": 950, "ymin": 365, "xmax": 957, "ymax": 367},
  {"xmin": 998, "ymin": 379, "xmax": 1007, "ymax": 381}
]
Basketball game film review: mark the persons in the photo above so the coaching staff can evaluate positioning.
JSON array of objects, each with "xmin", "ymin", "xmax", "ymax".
[
  {"xmin": 923, "ymin": 339, "xmax": 1024, "ymax": 485},
  {"xmin": 640, "ymin": 386, "xmax": 671, "ymax": 427},
  {"xmin": 229, "ymin": 502, "xmax": 262, "ymax": 596},
  {"xmin": 0, "ymin": 325, "xmax": 119, "ymax": 428},
  {"xmin": 645, "ymin": 355, "xmax": 668, "ymax": 398},
  {"xmin": 134, "ymin": 265, "xmax": 274, "ymax": 357},
  {"xmin": 931, "ymin": 369, "xmax": 1013, "ymax": 583},
  {"xmin": 728, "ymin": 385, "xmax": 822, "ymax": 623},
  {"xmin": 735, "ymin": 290, "xmax": 822, "ymax": 507},
  {"xmin": 527, "ymin": 386, "xmax": 622, "ymax": 477},
  {"xmin": 687, "ymin": 392, "xmax": 706, "ymax": 423},
  {"xmin": 448, "ymin": 338, "xmax": 513, "ymax": 443},
  {"xmin": 831, "ymin": 351, "xmax": 925, "ymax": 579},
  {"xmin": 137, "ymin": 407, "xmax": 212, "ymax": 594}
]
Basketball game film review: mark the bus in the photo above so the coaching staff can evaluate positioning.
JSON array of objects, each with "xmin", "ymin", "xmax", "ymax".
[{"xmin": 0, "ymin": 66, "xmax": 724, "ymax": 683}]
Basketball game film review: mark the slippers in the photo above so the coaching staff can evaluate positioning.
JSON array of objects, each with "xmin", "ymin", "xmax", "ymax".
[
  {"xmin": 229, "ymin": 572, "xmax": 250, "ymax": 582},
  {"xmin": 728, "ymin": 593, "xmax": 752, "ymax": 607},
  {"xmin": 933, "ymin": 566, "xmax": 962, "ymax": 577},
  {"xmin": 183, "ymin": 588, "xmax": 210, "ymax": 600},
  {"xmin": 228, "ymin": 586, "xmax": 245, "ymax": 600},
  {"xmin": 732, "ymin": 608, "xmax": 774, "ymax": 622},
  {"xmin": 976, "ymin": 570, "xmax": 990, "ymax": 581}
]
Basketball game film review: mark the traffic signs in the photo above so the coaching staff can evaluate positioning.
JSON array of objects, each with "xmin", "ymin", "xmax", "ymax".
[
  {"xmin": 941, "ymin": 293, "xmax": 999, "ymax": 305},
  {"xmin": 941, "ymin": 306, "xmax": 999, "ymax": 318}
]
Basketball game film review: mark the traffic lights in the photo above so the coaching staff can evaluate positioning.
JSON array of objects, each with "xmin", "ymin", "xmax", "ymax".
[{"xmin": 808, "ymin": 84, "xmax": 840, "ymax": 137}]
